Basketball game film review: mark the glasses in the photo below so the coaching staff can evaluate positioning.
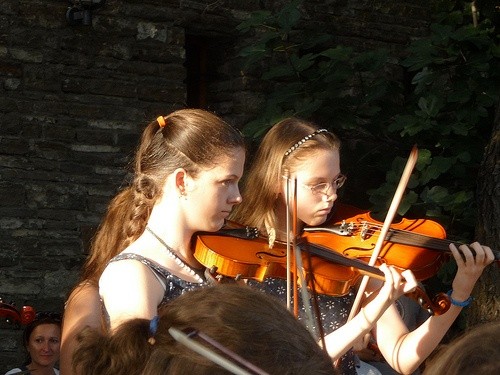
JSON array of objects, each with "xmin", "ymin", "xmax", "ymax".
[{"xmin": 282, "ymin": 173, "xmax": 347, "ymax": 196}]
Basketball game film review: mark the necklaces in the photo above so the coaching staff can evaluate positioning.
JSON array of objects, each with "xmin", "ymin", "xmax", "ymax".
[{"xmin": 144, "ymin": 226, "xmax": 206, "ymax": 282}]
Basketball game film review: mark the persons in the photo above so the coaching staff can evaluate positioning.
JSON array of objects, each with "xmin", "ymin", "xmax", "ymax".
[
  {"xmin": 59, "ymin": 109, "xmax": 500, "ymax": 375},
  {"xmin": 5, "ymin": 311, "xmax": 62, "ymax": 375}
]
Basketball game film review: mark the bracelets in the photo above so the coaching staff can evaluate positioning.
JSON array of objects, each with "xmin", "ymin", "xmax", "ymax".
[
  {"xmin": 447, "ymin": 290, "xmax": 473, "ymax": 307},
  {"xmin": 361, "ymin": 307, "xmax": 375, "ymax": 328}
]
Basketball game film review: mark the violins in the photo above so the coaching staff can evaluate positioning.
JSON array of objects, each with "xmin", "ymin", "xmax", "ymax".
[
  {"xmin": 192, "ymin": 219, "xmax": 452, "ymax": 318},
  {"xmin": 0, "ymin": 292, "xmax": 37, "ymax": 330},
  {"xmin": 298, "ymin": 202, "xmax": 500, "ymax": 283}
]
